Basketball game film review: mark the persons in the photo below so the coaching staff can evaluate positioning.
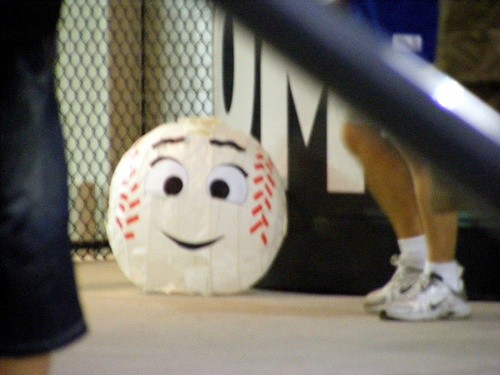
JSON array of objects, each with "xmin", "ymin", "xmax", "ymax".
[{"xmin": 329, "ymin": 0, "xmax": 473, "ymax": 321}]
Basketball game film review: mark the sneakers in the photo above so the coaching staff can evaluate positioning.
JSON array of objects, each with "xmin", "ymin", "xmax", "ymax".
[
  {"xmin": 382, "ymin": 266, "xmax": 471, "ymax": 321},
  {"xmin": 362, "ymin": 254, "xmax": 421, "ymax": 312}
]
